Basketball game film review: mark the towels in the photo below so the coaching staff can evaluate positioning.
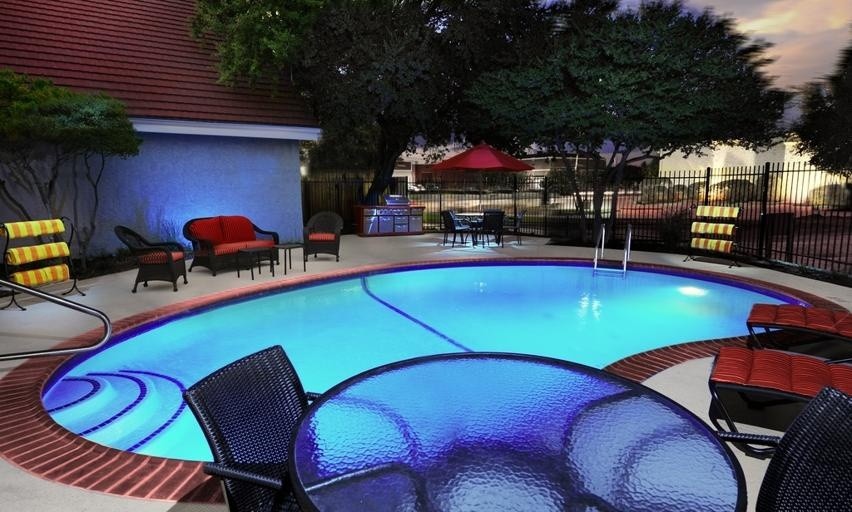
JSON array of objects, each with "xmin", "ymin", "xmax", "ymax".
[
  {"xmin": 690, "ymin": 205, "xmax": 740, "ymax": 255},
  {"xmin": 0, "ymin": 219, "xmax": 71, "ymax": 288}
]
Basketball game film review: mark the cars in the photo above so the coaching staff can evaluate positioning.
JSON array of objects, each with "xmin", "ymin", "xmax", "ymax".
[{"xmin": 401, "ymin": 182, "xmax": 440, "ymax": 194}]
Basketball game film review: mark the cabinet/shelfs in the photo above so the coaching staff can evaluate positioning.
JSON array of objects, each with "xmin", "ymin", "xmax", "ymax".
[{"xmin": 362, "ymin": 209, "xmax": 424, "ymax": 233}]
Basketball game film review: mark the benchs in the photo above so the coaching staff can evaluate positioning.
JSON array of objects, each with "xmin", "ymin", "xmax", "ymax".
[{"xmin": 708, "ymin": 302, "xmax": 851, "ymax": 458}]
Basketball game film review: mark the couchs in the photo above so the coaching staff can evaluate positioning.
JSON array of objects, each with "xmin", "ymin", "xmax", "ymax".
[{"xmin": 183, "ymin": 215, "xmax": 280, "ymax": 277}]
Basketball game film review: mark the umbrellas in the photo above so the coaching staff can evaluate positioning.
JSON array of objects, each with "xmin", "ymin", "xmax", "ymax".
[{"xmin": 432, "ymin": 144, "xmax": 536, "ymax": 214}]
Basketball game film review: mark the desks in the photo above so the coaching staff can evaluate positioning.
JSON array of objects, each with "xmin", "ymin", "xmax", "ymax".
[{"xmin": 286, "ymin": 350, "xmax": 749, "ymax": 510}]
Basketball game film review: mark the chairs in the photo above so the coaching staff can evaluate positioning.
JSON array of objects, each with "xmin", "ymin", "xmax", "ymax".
[
  {"xmin": 715, "ymin": 385, "xmax": 850, "ymax": 511},
  {"xmin": 115, "ymin": 224, "xmax": 188, "ymax": 294},
  {"xmin": 181, "ymin": 343, "xmax": 324, "ymax": 511},
  {"xmin": 440, "ymin": 208, "xmax": 527, "ymax": 249},
  {"xmin": 303, "ymin": 211, "xmax": 344, "ymax": 263}
]
